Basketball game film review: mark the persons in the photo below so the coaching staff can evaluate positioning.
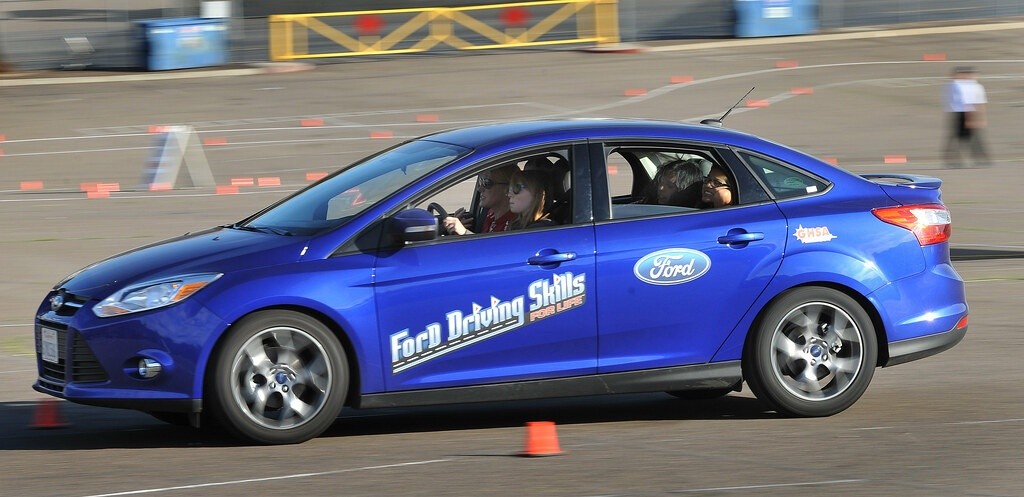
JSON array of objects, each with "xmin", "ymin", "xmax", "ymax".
[
  {"xmin": 683, "ymin": 162, "xmax": 734, "ymax": 209},
  {"xmin": 646, "ymin": 159, "xmax": 704, "ymax": 208},
  {"xmin": 940, "ymin": 65, "xmax": 995, "ymax": 176},
  {"xmin": 452, "ymin": 163, "xmax": 522, "ymax": 233},
  {"xmin": 442, "ymin": 170, "xmax": 563, "ymax": 238}
]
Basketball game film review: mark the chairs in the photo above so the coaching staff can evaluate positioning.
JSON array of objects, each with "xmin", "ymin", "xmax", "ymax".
[{"xmin": 524, "ymin": 156, "xmax": 573, "ymax": 227}]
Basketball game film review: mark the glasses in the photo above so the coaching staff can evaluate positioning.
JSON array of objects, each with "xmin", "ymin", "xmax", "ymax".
[
  {"xmin": 477, "ymin": 177, "xmax": 510, "ymax": 189},
  {"xmin": 508, "ymin": 184, "xmax": 530, "ymax": 194},
  {"xmin": 702, "ymin": 176, "xmax": 731, "ymax": 188}
]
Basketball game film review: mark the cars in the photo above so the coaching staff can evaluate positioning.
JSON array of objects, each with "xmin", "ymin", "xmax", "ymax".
[{"xmin": 30, "ymin": 119, "xmax": 968, "ymax": 446}]
